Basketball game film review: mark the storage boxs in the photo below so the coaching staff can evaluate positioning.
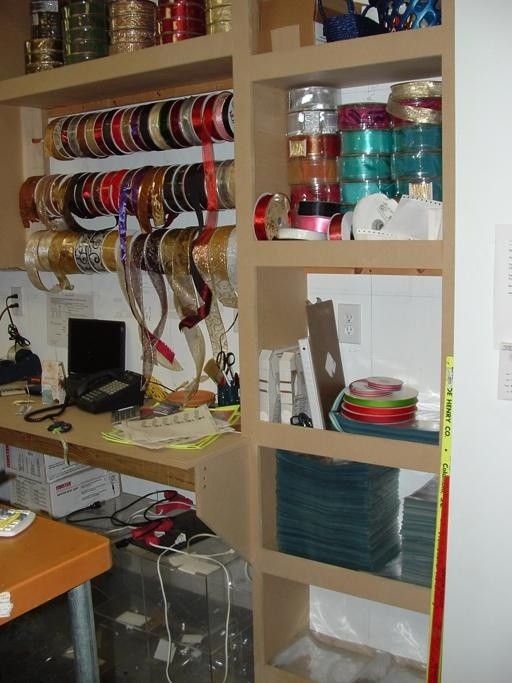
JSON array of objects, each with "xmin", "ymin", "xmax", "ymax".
[
  {"xmin": 0, "ymin": 440, "xmax": 95, "ymax": 480},
  {"xmin": 0, "ymin": 469, "xmax": 122, "ymax": 519}
]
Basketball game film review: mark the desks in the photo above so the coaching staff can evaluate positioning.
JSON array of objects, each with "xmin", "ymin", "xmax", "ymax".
[
  {"xmin": 0, "ymin": 501, "xmax": 115, "ymax": 683},
  {"xmin": 0, "ymin": 370, "xmax": 251, "ymax": 563}
]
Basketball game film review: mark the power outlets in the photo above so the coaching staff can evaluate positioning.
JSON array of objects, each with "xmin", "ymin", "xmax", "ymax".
[
  {"xmin": 9, "ymin": 286, "xmax": 23, "ymax": 316},
  {"xmin": 337, "ymin": 303, "xmax": 361, "ymax": 344}
]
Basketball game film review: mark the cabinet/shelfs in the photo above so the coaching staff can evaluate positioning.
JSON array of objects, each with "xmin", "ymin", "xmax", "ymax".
[
  {"xmin": 0, "ymin": 0, "xmax": 251, "ymax": 681},
  {"xmin": 237, "ymin": 0, "xmax": 451, "ymax": 683}
]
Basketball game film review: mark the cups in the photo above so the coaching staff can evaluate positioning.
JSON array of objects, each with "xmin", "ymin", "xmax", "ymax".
[{"xmin": 217, "ymin": 385, "xmax": 240, "ymax": 408}]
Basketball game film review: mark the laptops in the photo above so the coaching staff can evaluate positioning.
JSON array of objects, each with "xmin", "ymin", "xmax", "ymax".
[{"xmin": 25, "ymin": 317, "xmax": 126, "ymax": 399}]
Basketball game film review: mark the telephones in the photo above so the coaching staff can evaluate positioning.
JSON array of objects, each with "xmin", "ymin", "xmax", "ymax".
[{"xmin": 71, "ymin": 368, "xmax": 144, "ymax": 415}]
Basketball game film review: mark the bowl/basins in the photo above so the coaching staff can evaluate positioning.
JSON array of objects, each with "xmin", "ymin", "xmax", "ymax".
[{"xmin": 167, "ymin": 390, "xmax": 215, "ymax": 408}]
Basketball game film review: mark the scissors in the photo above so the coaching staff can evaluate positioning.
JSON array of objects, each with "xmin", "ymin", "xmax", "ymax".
[{"xmin": 216, "ymin": 352, "xmax": 235, "ymax": 376}]
